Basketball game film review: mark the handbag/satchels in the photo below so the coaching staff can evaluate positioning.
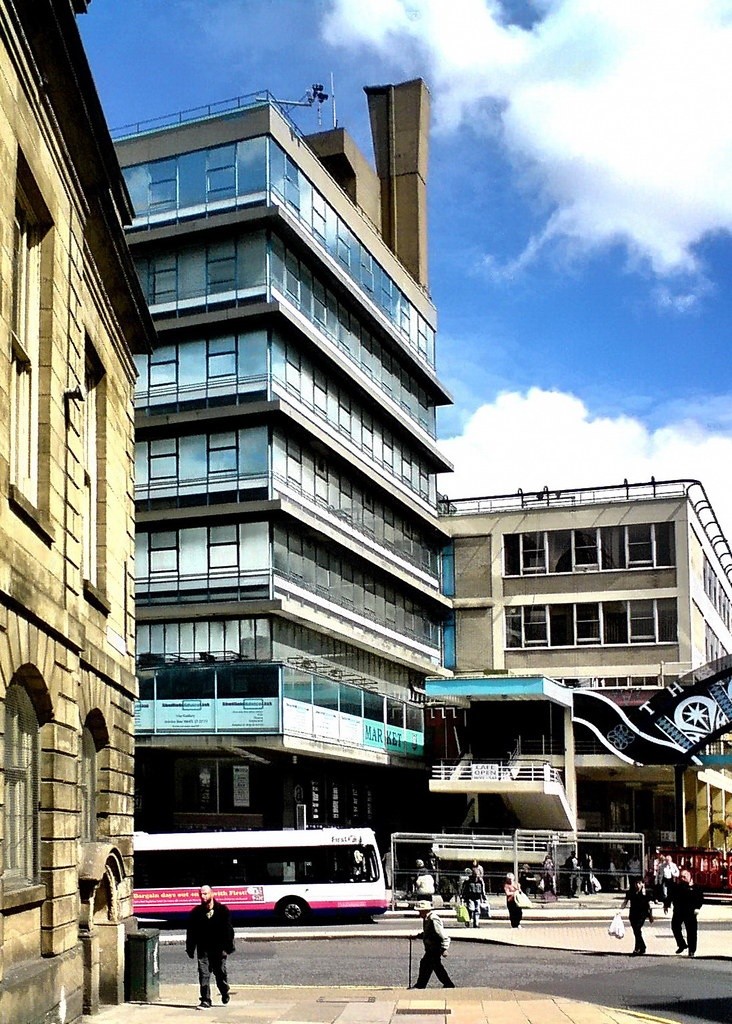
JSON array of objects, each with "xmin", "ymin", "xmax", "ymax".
[
  {"xmin": 479, "ymin": 900, "xmax": 490, "ymax": 919},
  {"xmin": 608, "ymin": 913, "xmax": 626, "ymax": 940},
  {"xmin": 456, "ymin": 905, "xmax": 471, "ymax": 923},
  {"xmin": 592, "ymin": 875, "xmax": 602, "ymax": 892},
  {"xmin": 514, "ymin": 888, "xmax": 533, "ymax": 908},
  {"xmin": 538, "ymin": 879, "xmax": 544, "ymax": 892}
]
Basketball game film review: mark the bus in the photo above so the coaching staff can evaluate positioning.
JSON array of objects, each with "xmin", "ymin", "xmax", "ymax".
[{"xmin": 133, "ymin": 828, "xmax": 387, "ymax": 924}]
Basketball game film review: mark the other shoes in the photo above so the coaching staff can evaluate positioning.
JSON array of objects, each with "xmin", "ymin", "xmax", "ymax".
[
  {"xmin": 676, "ymin": 946, "xmax": 689, "ymax": 953},
  {"xmin": 633, "ymin": 949, "xmax": 645, "ymax": 955},
  {"xmin": 198, "ymin": 1000, "xmax": 213, "ymax": 1010},
  {"xmin": 222, "ymin": 992, "xmax": 229, "ymax": 1004}
]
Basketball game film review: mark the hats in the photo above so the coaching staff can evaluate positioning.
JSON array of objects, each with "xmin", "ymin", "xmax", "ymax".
[
  {"xmin": 414, "ymin": 900, "xmax": 432, "ymax": 911},
  {"xmin": 416, "ymin": 860, "xmax": 425, "ymax": 867}
]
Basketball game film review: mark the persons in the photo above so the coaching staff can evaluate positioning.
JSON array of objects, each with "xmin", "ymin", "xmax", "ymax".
[
  {"xmin": 381, "ymin": 845, "xmax": 680, "ymax": 929},
  {"xmin": 407, "ymin": 901, "xmax": 456, "ymax": 989},
  {"xmin": 664, "ymin": 869, "xmax": 705, "ymax": 957},
  {"xmin": 621, "ymin": 876, "xmax": 653, "ymax": 957},
  {"xmin": 186, "ymin": 884, "xmax": 237, "ymax": 1010}
]
